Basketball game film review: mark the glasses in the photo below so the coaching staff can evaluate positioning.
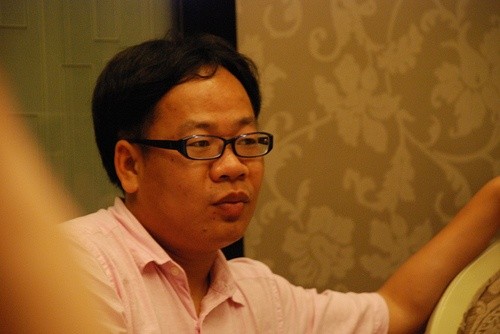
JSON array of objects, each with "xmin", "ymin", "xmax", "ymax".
[{"xmin": 124, "ymin": 131, "xmax": 273, "ymax": 161}]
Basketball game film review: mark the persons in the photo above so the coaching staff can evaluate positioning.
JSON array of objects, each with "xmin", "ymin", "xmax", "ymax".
[{"xmin": 40, "ymin": 31, "xmax": 500, "ymax": 334}]
